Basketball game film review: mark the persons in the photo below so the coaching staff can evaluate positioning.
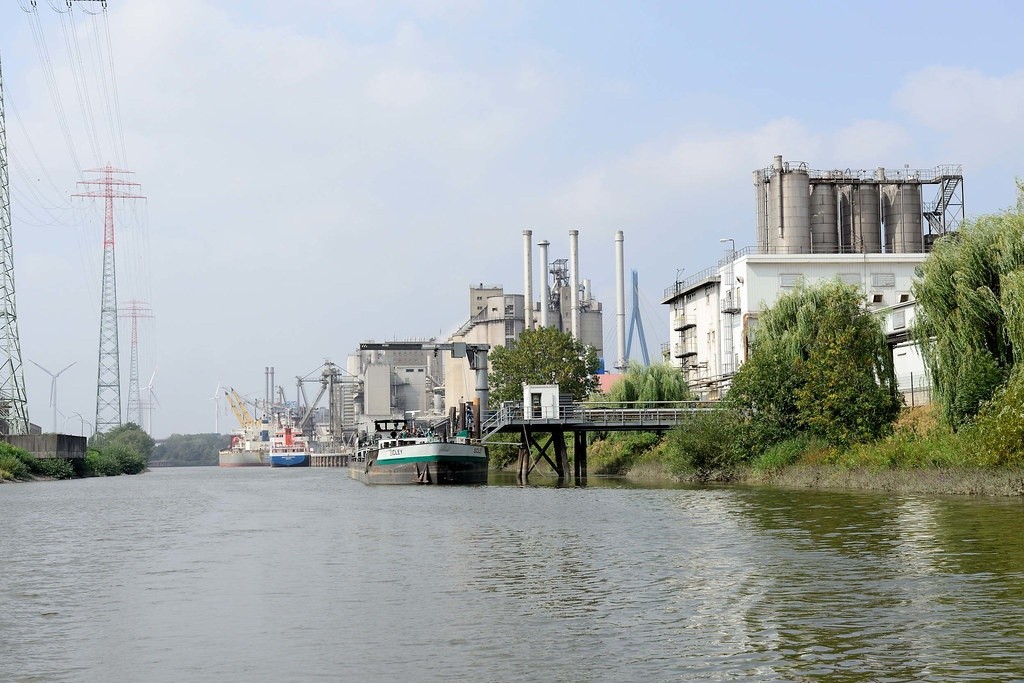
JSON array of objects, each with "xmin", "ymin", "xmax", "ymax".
[{"xmin": 375, "ymin": 423, "xmax": 432, "ymax": 445}]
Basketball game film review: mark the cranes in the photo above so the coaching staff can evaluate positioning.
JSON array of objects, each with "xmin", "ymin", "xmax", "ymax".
[{"xmin": 226, "ymin": 356, "xmax": 366, "ymax": 454}]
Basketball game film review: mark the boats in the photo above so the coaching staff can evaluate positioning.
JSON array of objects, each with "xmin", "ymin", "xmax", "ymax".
[
  {"xmin": 346, "ymin": 400, "xmax": 490, "ymax": 486},
  {"xmin": 269, "ymin": 422, "xmax": 312, "ymax": 468}
]
